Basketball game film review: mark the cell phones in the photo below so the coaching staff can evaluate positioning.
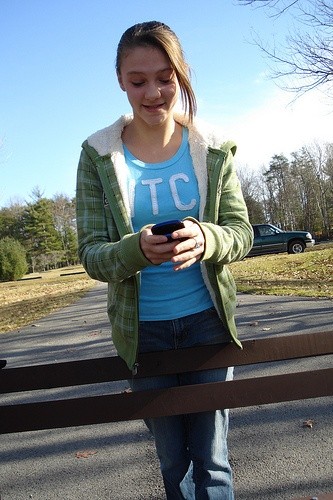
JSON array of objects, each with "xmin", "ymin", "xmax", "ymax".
[{"xmin": 150, "ymin": 220, "xmax": 189, "ymax": 243}]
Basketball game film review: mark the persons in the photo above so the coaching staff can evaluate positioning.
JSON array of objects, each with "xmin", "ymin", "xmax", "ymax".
[{"xmin": 76, "ymin": 21, "xmax": 253, "ymax": 500}]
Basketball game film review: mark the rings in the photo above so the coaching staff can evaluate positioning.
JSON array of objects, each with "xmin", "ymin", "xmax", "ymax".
[{"xmin": 193, "ymin": 237, "xmax": 200, "ymax": 249}]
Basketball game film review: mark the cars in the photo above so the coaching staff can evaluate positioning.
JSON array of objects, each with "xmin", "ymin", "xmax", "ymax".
[{"xmin": 243, "ymin": 222, "xmax": 315, "ymax": 258}]
